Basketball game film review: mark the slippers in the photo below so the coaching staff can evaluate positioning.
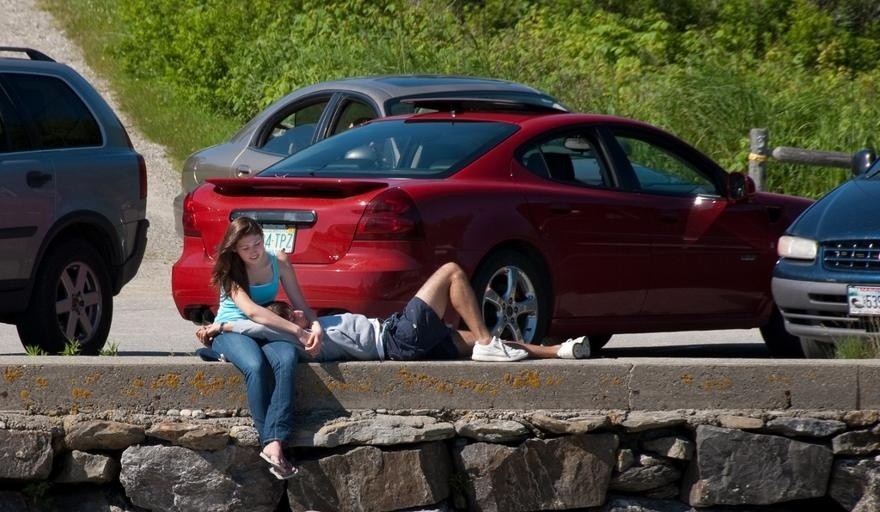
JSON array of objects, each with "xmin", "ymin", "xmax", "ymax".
[{"xmin": 260, "ymin": 452, "xmax": 298, "ymax": 480}]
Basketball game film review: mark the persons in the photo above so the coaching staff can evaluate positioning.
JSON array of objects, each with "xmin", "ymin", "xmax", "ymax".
[
  {"xmin": 195, "ymin": 261, "xmax": 591, "ymax": 363},
  {"xmin": 211, "ymin": 216, "xmax": 324, "ymax": 480}
]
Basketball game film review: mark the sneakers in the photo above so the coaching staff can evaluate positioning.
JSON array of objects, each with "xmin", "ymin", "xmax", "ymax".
[
  {"xmin": 557, "ymin": 336, "xmax": 590, "ymax": 358},
  {"xmin": 472, "ymin": 336, "xmax": 528, "ymax": 362}
]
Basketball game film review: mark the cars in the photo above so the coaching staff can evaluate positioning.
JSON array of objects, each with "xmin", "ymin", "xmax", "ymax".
[
  {"xmin": 175, "ymin": 74, "xmax": 707, "ymax": 242},
  {"xmin": 771, "ymin": 149, "xmax": 875, "ymax": 358},
  {"xmin": 172, "ymin": 98, "xmax": 816, "ymax": 356}
]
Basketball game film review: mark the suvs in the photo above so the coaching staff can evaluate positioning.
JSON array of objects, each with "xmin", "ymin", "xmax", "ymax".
[{"xmin": 0, "ymin": 46, "xmax": 149, "ymax": 356}]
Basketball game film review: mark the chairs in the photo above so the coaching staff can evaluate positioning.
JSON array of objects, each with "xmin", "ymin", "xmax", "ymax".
[{"xmin": 527, "ymin": 151, "xmax": 592, "ymax": 187}]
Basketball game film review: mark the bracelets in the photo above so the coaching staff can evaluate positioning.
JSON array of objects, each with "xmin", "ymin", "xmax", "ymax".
[{"xmin": 219, "ymin": 322, "xmax": 226, "ymax": 335}]
